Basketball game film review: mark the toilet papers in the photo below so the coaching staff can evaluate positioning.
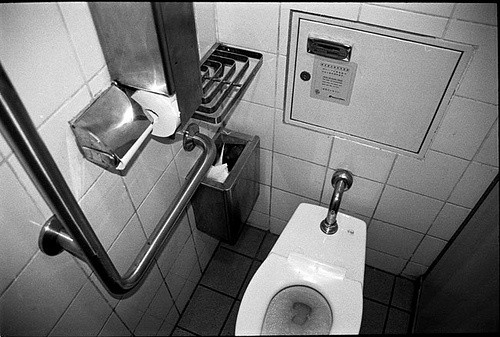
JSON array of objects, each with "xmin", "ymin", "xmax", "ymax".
[
  {"xmin": 130, "ymin": 90, "xmax": 181, "ymax": 138},
  {"xmin": 69, "ymin": 84, "xmax": 154, "ymax": 176}
]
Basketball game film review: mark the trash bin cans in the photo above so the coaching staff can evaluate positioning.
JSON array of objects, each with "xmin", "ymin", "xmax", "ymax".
[{"xmin": 186, "ymin": 127, "xmax": 260, "ymax": 246}]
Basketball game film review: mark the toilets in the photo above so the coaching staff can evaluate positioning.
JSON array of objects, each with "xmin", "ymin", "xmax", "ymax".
[{"xmin": 234, "ymin": 202, "xmax": 368, "ymax": 336}]
